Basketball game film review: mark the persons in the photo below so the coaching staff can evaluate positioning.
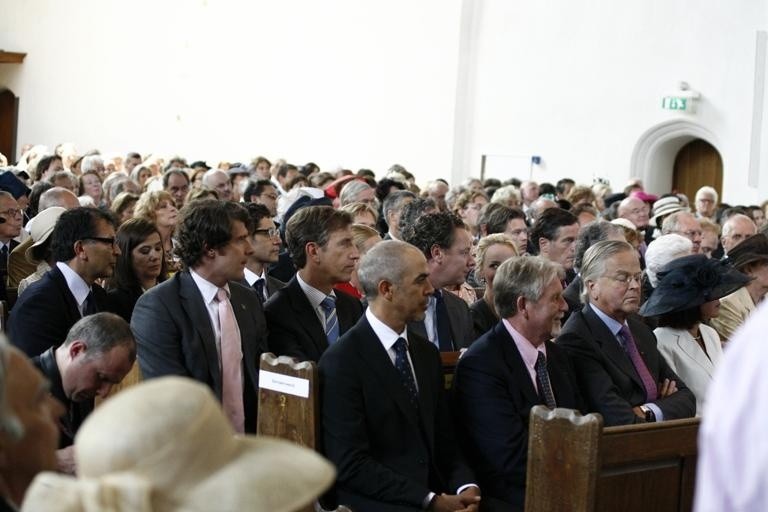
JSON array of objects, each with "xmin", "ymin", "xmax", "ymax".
[{"xmin": 1, "ymin": 146, "xmax": 767, "ymax": 512}]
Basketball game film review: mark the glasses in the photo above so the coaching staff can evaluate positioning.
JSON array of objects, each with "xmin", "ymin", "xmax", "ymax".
[
  {"xmin": 254, "ymin": 228, "xmax": 281, "ymax": 239},
  {"xmin": 81, "ymin": 236, "xmax": 120, "ymax": 247},
  {"xmin": 600, "ymin": 274, "xmax": 647, "ymax": 286}
]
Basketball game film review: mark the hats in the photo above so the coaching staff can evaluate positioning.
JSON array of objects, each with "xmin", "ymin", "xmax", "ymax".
[
  {"xmin": 721, "ymin": 232, "xmax": 767, "ymax": 270},
  {"xmin": 638, "ymin": 253, "xmax": 757, "ymax": 318},
  {"xmin": 225, "ymin": 163, "xmax": 369, "ymax": 229},
  {"xmin": 648, "ymin": 197, "xmax": 691, "ymax": 227},
  {"xmin": 18, "ymin": 375, "xmax": 338, "ymax": 511},
  {"xmin": 24, "ymin": 206, "xmax": 68, "ymax": 265}
]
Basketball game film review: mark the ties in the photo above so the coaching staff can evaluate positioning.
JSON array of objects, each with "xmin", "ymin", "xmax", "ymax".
[
  {"xmin": 211, "ymin": 288, "xmax": 246, "ymax": 434},
  {"xmin": 392, "ymin": 337, "xmax": 418, "ymax": 396},
  {"xmin": 534, "ymin": 350, "xmax": 555, "ymax": 409},
  {"xmin": 319, "ymin": 297, "xmax": 341, "ymax": 345},
  {"xmin": 434, "ymin": 286, "xmax": 453, "ymax": 353},
  {"xmin": 619, "ymin": 322, "xmax": 660, "ymax": 402}
]
